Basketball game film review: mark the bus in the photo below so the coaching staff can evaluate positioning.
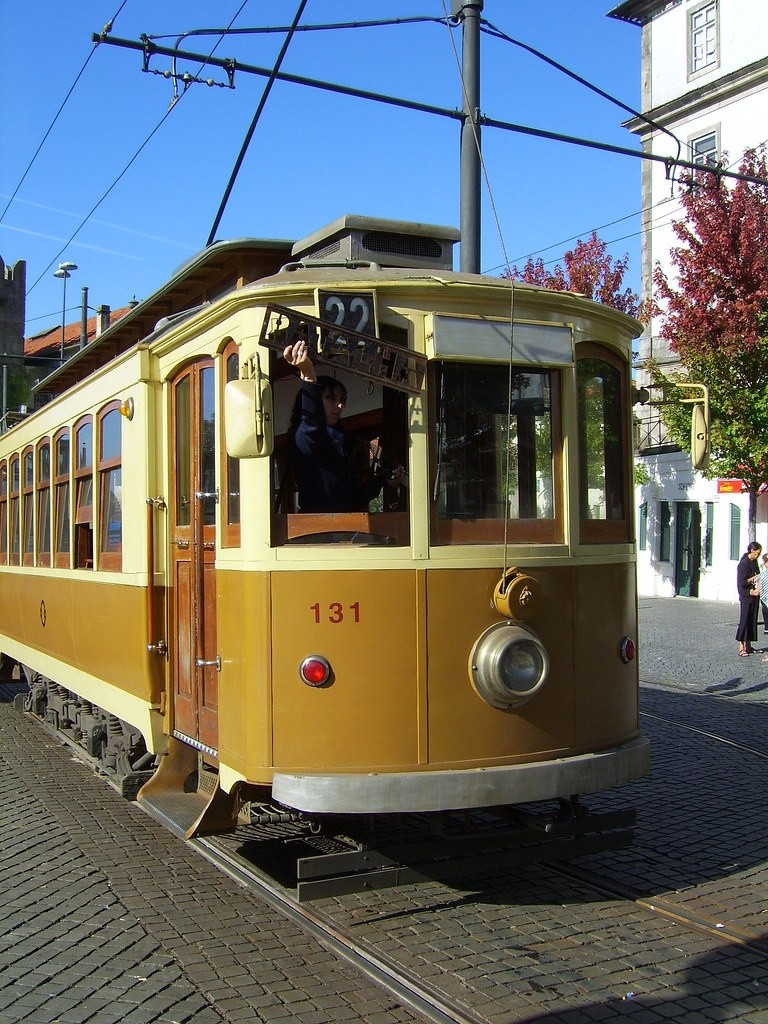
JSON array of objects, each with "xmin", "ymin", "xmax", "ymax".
[{"xmin": 0, "ymin": 209, "xmax": 712, "ymax": 904}]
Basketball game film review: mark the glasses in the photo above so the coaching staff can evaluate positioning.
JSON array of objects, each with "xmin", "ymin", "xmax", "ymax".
[{"xmin": 324, "ymin": 392, "xmax": 347, "ymax": 405}]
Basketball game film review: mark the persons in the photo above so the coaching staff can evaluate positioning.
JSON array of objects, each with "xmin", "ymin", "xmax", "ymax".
[
  {"xmin": 735, "ymin": 541, "xmax": 768, "ymax": 658},
  {"xmin": 282, "ymin": 340, "xmax": 384, "ymax": 512}
]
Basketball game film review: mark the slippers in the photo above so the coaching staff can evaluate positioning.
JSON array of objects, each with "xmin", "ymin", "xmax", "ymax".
[
  {"xmin": 747, "ymin": 647, "xmax": 758, "ymax": 653},
  {"xmin": 738, "ymin": 650, "xmax": 749, "ymax": 657}
]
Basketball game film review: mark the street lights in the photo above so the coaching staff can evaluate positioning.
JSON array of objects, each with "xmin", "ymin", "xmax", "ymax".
[{"xmin": 52, "ymin": 260, "xmax": 78, "ymax": 367}]
